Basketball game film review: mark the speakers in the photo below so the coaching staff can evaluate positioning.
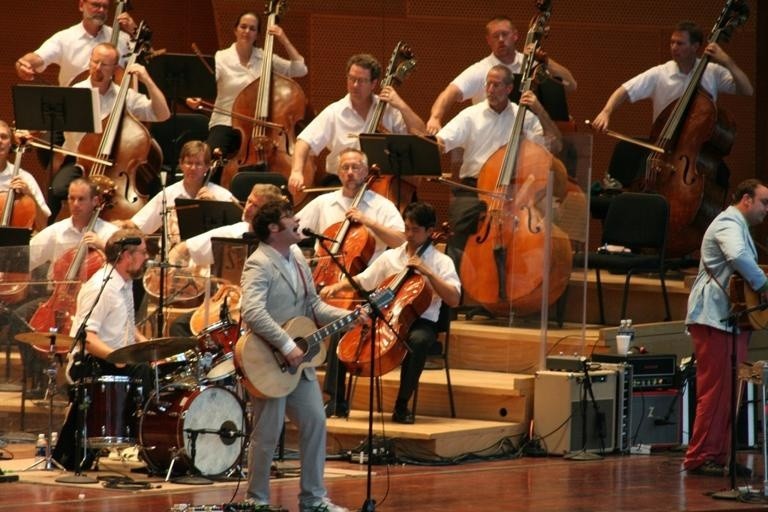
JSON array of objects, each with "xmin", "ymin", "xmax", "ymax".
[
  {"xmin": 534, "ymin": 370, "xmax": 617, "ymax": 453},
  {"xmin": 630, "ymin": 390, "xmax": 683, "ymax": 452}
]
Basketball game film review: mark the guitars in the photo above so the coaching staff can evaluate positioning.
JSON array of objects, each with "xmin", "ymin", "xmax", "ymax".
[
  {"xmin": 233, "ymin": 286, "xmax": 395, "ymax": 399},
  {"xmin": 728, "ymin": 267, "xmax": 767, "ymax": 332}
]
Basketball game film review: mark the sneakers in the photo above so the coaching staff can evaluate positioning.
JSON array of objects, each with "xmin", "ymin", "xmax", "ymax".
[
  {"xmin": 323, "ymin": 398, "xmax": 349, "ymax": 417},
  {"xmin": 299, "ymin": 496, "xmax": 349, "ymax": 512},
  {"xmin": 392, "ymin": 410, "xmax": 415, "ymax": 424},
  {"xmin": 24, "ymin": 382, "xmax": 47, "ymax": 399},
  {"xmin": 687, "ymin": 459, "xmax": 751, "ymax": 478}
]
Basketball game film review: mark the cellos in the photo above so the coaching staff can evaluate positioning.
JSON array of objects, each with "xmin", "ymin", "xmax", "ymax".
[
  {"xmin": 221, "ymin": 0, "xmax": 315, "ymax": 209},
  {"xmin": 355, "ymin": 38, "xmax": 418, "ymax": 220},
  {"xmin": 460, "ymin": 0, "xmax": 573, "ymax": 315},
  {"xmin": 336, "ymin": 222, "xmax": 456, "ymax": 377},
  {"xmin": 142, "ymin": 148, "xmax": 225, "ymax": 307},
  {"xmin": 50, "ymin": 0, "xmax": 164, "ymax": 227},
  {"xmin": 1, "ymin": 135, "xmax": 38, "ymax": 300},
  {"xmin": 25, "ymin": 177, "xmax": 116, "ymax": 356},
  {"xmin": 310, "ymin": 163, "xmax": 381, "ymax": 325},
  {"xmin": 632, "ymin": 2, "xmax": 751, "ymax": 254}
]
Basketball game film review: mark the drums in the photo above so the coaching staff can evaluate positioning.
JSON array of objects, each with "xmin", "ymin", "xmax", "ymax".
[
  {"xmin": 80, "ymin": 375, "xmax": 143, "ymax": 449},
  {"xmin": 198, "ymin": 323, "xmax": 240, "ymax": 356},
  {"xmin": 140, "ymin": 383, "xmax": 250, "ymax": 479},
  {"xmin": 210, "ymin": 359, "xmax": 247, "ymax": 398}
]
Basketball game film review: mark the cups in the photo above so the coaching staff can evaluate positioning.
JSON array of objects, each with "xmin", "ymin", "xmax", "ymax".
[{"xmin": 616, "ymin": 336, "xmax": 630, "ymax": 355}]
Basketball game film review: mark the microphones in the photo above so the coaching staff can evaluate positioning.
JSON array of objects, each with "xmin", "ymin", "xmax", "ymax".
[
  {"xmin": 144, "ymin": 259, "xmax": 182, "ymax": 270},
  {"xmin": 301, "ymin": 226, "xmax": 338, "ymax": 245},
  {"xmin": 112, "ymin": 236, "xmax": 143, "ymax": 246}
]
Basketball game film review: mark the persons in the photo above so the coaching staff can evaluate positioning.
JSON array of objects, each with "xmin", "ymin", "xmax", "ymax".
[
  {"xmin": 292, "ymin": 146, "xmax": 407, "ymax": 269},
  {"xmin": 239, "ymin": 197, "xmax": 368, "ymax": 511},
  {"xmin": 318, "ymin": 200, "xmax": 461, "ymax": 423},
  {"xmin": 125, "ymin": 140, "xmax": 240, "ymax": 249},
  {"xmin": 435, "ymin": 62, "xmax": 562, "ymax": 270},
  {"xmin": 425, "ymin": 14, "xmax": 578, "ymax": 136},
  {"xmin": 167, "ymin": 182, "xmax": 292, "ymax": 338},
  {"xmin": 287, "ymin": 51, "xmax": 430, "ymax": 197},
  {"xmin": 47, "ymin": 41, "xmax": 171, "ymax": 227},
  {"xmin": 9, "ymin": 175, "xmax": 119, "ymax": 400},
  {"xmin": 0, "ymin": 120, "xmax": 53, "ymax": 233},
  {"xmin": 590, "ymin": 20, "xmax": 754, "ymax": 200},
  {"xmin": 14, "ymin": 1, "xmax": 138, "ymax": 88},
  {"xmin": 179, "ymin": 8, "xmax": 309, "ymax": 159},
  {"xmin": 64, "ymin": 229, "xmax": 160, "ymax": 434},
  {"xmin": 681, "ymin": 179, "xmax": 768, "ymax": 477}
]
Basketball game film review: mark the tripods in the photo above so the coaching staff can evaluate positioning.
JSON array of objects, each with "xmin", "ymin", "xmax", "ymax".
[{"xmin": 19, "ymin": 384, "xmax": 67, "ymax": 473}]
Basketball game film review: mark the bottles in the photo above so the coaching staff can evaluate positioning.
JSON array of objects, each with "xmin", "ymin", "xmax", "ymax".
[
  {"xmin": 618, "ymin": 319, "xmax": 635, "ymax": 348},
  {"xmin": 49, "ymin": 431, "xmax": 59, "ymax": 459},
  {"xmin": 35, "ymin": 433, "xmax": 47, "ymax": 471}
]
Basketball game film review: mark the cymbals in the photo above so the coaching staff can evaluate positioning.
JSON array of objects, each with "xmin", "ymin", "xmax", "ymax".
[
  {"xmin": 109, "ymin": 337, "xmax": 199, "ymax": 365},
  {"xmin": 14, "ymin": 331, "xmax": 88, "ymax": 349}
]
